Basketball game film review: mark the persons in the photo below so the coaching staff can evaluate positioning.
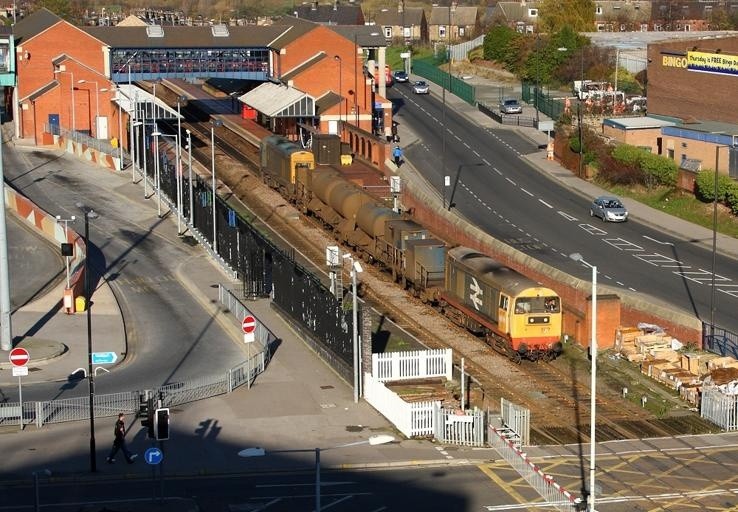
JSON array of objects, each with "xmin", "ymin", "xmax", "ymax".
[
  {"xmin": 547, "ymin": 300, "xmax": 554, "ymax": 311},
  {"xmin": 563, "ymin": 82, "xmax": 642, "ymax": 116},
  {"xmin": 613, "ymin": 203, "xmax": 621, "ymax": 208},
  {"xmin": 105, "ymin": 412, "xmax": 138, "ymax": 461},
  {"xmin": 108, "ymin": 421, "xmax": 135, "ymax": 464},
  {"xmin": 392, "ymin": 147, "xmax": 402, "ymax": 164},
  {"xmin": 607, "ymin": 201, "xmax": 614, "ymax": 208}
]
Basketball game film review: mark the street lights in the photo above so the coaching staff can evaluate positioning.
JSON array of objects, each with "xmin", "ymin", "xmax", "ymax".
[
  {"xmin": 131, "ymin": 121, "xmax": 161, "ymax": 218},
  {"xmin": 151, "ymin": 132, "xmax": 181, "ymax": 234},
  {"xmin": 75, "ymin": 200, "xmax": 99, "ymax": 475},
  {"xmin": 570, "ymin": 239, "xmax": 598, "ymax": 507},
  {"xmin": 343, "ymin": 251, "xmax": 363, "ymax": 403},
  {"xmin": 54, "ymin": 69, "xmax": 124, "ymax": 170},
  {"xmin": 123, "ymin": 108, "xmax": 149, "ymax": 199},
  {"xmin": 238, "ymin": 434, "xmax": 394, "ymax": 512},
  {"xmin": 110, "ymin": 97, "xmax": 135, "ymax": 185},
  {"xmin": 555, "ymin": 46, "xmax": 583, "ymax": 175}
]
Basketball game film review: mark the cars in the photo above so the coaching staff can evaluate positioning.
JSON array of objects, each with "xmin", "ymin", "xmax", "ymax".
[
  {"xmin": 393, "ymin": 71, "xmax": 430, "ymax": 94},
  {"xmin": 590, "ymin": 195, "xmax": 629, "ymax": 223},
  {"xmin": 500, "ymin": 97, "xmax": 522, "ymax": 113},
  {"xmin": 574, "ymin": 80, "xmax": 647, "ymax": 111}
]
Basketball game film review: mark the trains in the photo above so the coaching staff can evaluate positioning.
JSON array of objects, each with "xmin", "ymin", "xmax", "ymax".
[{"xmin": 259, "ymin": 135, "xmax": 562, "ymax": 364}]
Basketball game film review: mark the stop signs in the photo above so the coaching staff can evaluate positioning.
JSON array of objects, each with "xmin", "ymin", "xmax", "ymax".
[
  {"xmin": 242, "ymin": 315, "xmax": 257, "ymax": 334},
  {"xmin": 8, "ymin": 348, "xmax": 30, "ymax": 367}
]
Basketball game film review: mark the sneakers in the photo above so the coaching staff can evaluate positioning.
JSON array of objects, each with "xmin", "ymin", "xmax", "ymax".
[{"xmin": 107, "ymin": 458, "xmax": 136, "ymax": 464}]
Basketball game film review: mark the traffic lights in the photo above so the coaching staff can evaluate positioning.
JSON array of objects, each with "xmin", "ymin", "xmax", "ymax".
[{"xmin": 139, "ymin": 397, "xmax": 171, "ymax": 442}]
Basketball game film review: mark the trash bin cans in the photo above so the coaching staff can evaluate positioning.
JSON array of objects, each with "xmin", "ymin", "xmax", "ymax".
[{"xmin": 75, "ymin": 295, "xmax": 87, "ymax": 312}]
